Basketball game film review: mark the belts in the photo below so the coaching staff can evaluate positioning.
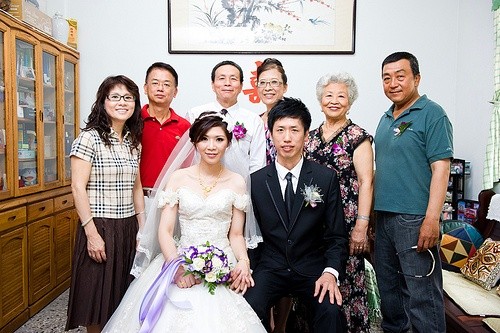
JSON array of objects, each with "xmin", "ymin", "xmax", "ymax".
[{"xmin": 143, "ymin": 189, "xmax": 156, "ymax": 196}]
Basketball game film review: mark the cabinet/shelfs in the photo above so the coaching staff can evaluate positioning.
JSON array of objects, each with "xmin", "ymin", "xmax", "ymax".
[{"xmin": 0, "ymin": 9, "xmax": 80, "ymax": 333}]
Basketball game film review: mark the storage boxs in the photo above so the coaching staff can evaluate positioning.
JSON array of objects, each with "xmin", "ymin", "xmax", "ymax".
[{"xmin": 8, "ymin": 0, "xmax": 53, "ymax": 36}]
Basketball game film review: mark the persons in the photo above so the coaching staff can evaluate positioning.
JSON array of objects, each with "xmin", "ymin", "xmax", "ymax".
[
  {"xmin": 372, "ymin": 51, "xmax": 454, "ymax": 333},
  {"xmin": 302, "ymin": 68, "xmax": 374, "ymax": 332},
  {"xmin": 239, "ymin": 95, "xmax": 348, "ymax": 332},
  {"xmin": 133, "ymin": 62, "xmax": 193, "ymax": 234},
  {"xmin": 192, "ymin": 58, "xmax": 269, "ymax": 209},
  {"xmin": 65, "ymin": 74, "xmax": 145, "ymax": 333},
  {"xmin": 100, "ymin": 107, "xmax": 268, "ymax": 332},
  {"xmin": 250, "ymin": 57, "xmax": 301, "ymax": 166}
]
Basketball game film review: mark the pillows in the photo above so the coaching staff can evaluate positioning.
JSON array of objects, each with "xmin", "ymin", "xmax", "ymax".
[
  {"xmin": 461, "ymin": 236, "xmax": 500, "ymax": 292},
  {"xmin": 438, "ymin": 219, "xmax": 484, "ymax": 275}
]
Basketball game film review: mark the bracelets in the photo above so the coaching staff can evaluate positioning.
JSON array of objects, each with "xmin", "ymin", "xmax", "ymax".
[{"xmin": 81, "ymin": 215, "xmax": 93, "ymax": 226}]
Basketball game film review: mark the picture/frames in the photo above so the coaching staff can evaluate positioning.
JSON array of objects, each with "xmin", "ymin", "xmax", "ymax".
[{"xmin": 166, "ymin": 0, "xmax": 357, "ymax": 57}]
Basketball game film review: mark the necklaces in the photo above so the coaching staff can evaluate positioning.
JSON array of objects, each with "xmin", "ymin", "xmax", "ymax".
[{"xmin": 197, "ymin": 162, "xmax": 223, "ymax": 193}]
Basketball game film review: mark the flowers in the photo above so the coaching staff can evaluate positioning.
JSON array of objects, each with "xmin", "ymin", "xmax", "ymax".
[
  {"xmin": 331, "ymin": 136, "xmax": 349, "ymax": 159},
  {"xmin": 181, "ymin": 241, "xmax": 238, "ymax": 294},
  {"xmin": 393, "ymin": 121, "xmax": 413, "ymax": 137},
  {"xmin": 233, "ymin": 120, "xmax": 248, "ymax": 140},
  {"xmin": 300, "ymin": 183, "xmax": 326, "ymax": 207}
]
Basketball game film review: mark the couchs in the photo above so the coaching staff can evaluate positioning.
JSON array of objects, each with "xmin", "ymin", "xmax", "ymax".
[{"xmin": 440, "ymin": 188, "xmax": 500, "ymax": 333}]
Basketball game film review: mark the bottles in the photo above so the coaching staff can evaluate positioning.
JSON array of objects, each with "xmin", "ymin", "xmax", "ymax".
[{"xmin": 52, "ymin": 9, "xmax": 70, "ymax": 44}]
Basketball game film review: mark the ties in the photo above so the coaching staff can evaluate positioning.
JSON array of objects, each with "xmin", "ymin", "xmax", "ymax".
[{"xmin": 285, "ymin": 172, "xmax": 295, "ymax": 224}]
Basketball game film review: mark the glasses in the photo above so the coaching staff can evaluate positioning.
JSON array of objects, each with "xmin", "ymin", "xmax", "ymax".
[
  {"xmin": 256, "ymin": 80, "xmax": 283, "ymax": 87},
  {"xmin": 106, "ymin": 93, "xmax": 137, "ymax": 102}
]
